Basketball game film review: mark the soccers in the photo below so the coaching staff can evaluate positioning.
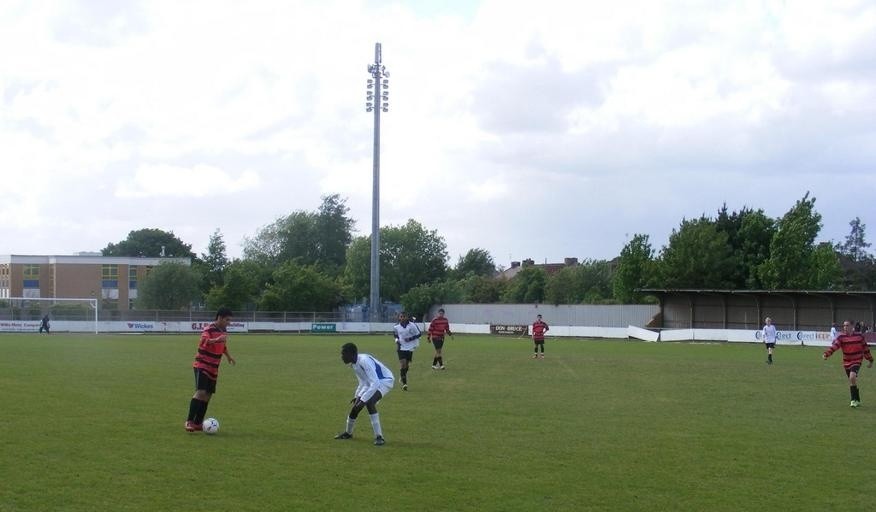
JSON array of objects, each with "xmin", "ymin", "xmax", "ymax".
[{"xmin": 202, "ymin": 418, "xmax": 219, "ymax": 434}]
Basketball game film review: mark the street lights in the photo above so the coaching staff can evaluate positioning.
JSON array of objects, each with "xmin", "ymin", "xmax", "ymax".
[{"xmin": 361, "ymin": 41, "xmax": 391, "ymax": 323}]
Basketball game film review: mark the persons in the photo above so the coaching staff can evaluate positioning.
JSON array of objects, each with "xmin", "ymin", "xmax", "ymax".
[
  {"xmin": 334, "ymin": 342, "xmax": 395, "ymax": 446},
  {"xmin": 182, "ymin": 306, "xmax": 236, "ymax": 434},
  {"xmin": 830, "ymin": 319, "xmax": 869, "ymax": 346},
  {"xmin": 532, "ymin": 314, "xmax": 549, "ymax": 359},
  {"xmin": 822, "ymin": 319, "xmax": 874, "ymax": 409},
  {"xmin": 426, "ymin": 308, "xmax": 454, "ymax": 370},
  {"xmin": 393, "ymin": 311, "xmax": 421, "ymax": 390},
  {"xmin": 38, "ymin": 314, "xmax": 50, "ymax": 334},
  {"xmin": 762, "ymin": 316, "xmax": 777, "ymax": 364}
]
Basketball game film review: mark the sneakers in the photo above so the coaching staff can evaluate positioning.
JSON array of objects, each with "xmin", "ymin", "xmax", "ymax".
[
  {"xmin": 334, "ymin": 431, "xmax": 353, "ymax": 439},
  {"xmin": 185, "ymin": 419, "xmax": 203, "ymax": 432},
  {"xmin": 431, "ymin": 365, "xmax": 445, "ymax": 370},
  {"xmin": 532, "ymin": 353, "xmax": 544, "ymax": 359},
  {"xmin": 374, "ymin": 436, "xmax": 385, "ymax": 445},
  {"xmin": 767, "ymin": 359, "xmax": 772, "ymax": 364},
  {"xmin": 849, "ymin": 399, "xmax": 860, "ymax": 407},
  {"xmin": 399, "ymin": 377, "xmax": 408, "ymax": 391}
]
